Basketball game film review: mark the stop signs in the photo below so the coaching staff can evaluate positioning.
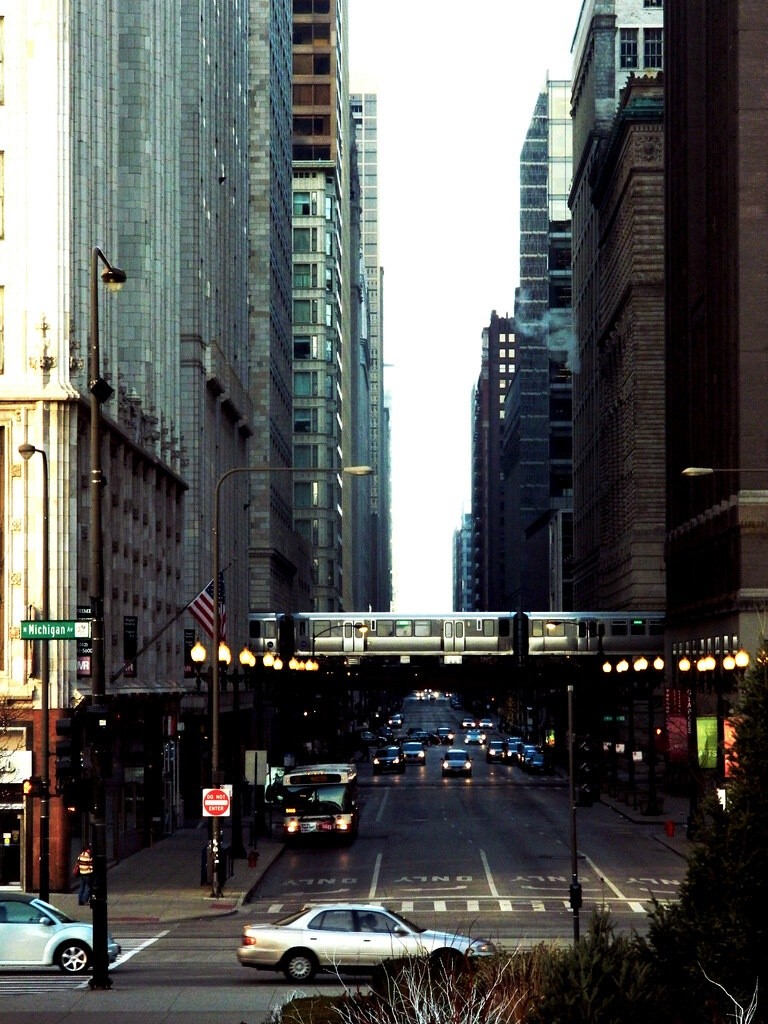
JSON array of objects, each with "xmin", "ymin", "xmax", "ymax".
[{"xmin": 204, "ymin": 790, "xmax": 229, "ymax": 815}]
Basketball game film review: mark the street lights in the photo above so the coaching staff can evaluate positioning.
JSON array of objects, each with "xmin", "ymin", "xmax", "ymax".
[
  {"xmin": 88, "ymin": 245, "xmax": 128, "ymax": 989},
  {"xmin": 603, "ymin": 655, "xmax": 664, "ymax": 817},
  {"xmin": 190, "ymin": 640, "xmax": 285, "ymax": 886},
  {"xmin": 679, "ymin": 648, "xmax": 750, "ymax": 842},
  {"xmin": 208, "ymin": 466, "xmax": 375, "ymax": 898},
  {"xmin": 545, "ymin": 620, "xmax": 604, "ymax": 654},
  {"xmin": 18, "ymin": 443, "xmax": 53, "ymax": 902},
  {"xmin": 311, "ymin": 623, "xmax": 368, "ymax": 664}
]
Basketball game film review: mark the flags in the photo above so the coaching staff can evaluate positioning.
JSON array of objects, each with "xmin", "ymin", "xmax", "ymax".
[{"xmin": 187, "ymin": 572, "xmax": 226, "ymax": 645}]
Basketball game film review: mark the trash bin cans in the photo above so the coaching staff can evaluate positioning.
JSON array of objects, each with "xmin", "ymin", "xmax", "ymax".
[
  {"xmin": 575, "ymin": 784, "xmax": 593, "ymax": 807},
  {"xmin": 200, "ymin": 841, "xmax": 231, "ymax": 883}
]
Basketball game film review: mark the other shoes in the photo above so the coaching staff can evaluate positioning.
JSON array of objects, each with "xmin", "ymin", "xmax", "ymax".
[{"xmin": 79, "ymin": 901, "xmax": 85, "ymax": 906}]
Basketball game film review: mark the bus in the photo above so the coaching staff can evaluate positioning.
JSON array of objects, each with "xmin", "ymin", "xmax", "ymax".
[{"xmin": 283, "ymin": 763, "xmax": 362, "ymax": 834}]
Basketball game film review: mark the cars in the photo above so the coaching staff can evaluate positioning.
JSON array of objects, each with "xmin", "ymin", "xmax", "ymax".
[
  {"xmin": 372, "ymin": 712, "xmax": 455, "ymax": 773},
  {"xmin": 0, "ymin": 891, "xmax": 122, "ymax": 975},
  {"xmin": 484, "ymin": 736, "xmax": 548, "ymax": 775},
  {"xmin": 235, "ymin": 903, "xmax": 499, "ymax": 988},
  {"xmin": 448, "ymin": 695, "xmax": 465, "ymax": 711},
  {"xmin": 441, "ymin": 749, "xmax": 474, "ymax": 778},
  {"xmin": 459, "ymin": 717, "xmax": 494, "ymax": 745}
]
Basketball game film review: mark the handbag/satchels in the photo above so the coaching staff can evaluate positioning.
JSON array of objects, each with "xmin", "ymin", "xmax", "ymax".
[{"xmin": 72, "ymin": 856, "xmax": 80, "ymax": 876}]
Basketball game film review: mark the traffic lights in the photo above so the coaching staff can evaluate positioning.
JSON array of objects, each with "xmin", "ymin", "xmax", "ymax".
[{"xmin": 22, "ymin": 780, "xmax": 42, "ymax": 795}]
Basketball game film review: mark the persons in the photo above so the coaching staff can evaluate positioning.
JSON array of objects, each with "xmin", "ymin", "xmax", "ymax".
[
  {"xmin": 76, "ymin": 844, "xmax": 93, "ymax": 905},
  {"xmin": 273, "ymin": 773, "xmax": 284, "ymax": 782}
]
